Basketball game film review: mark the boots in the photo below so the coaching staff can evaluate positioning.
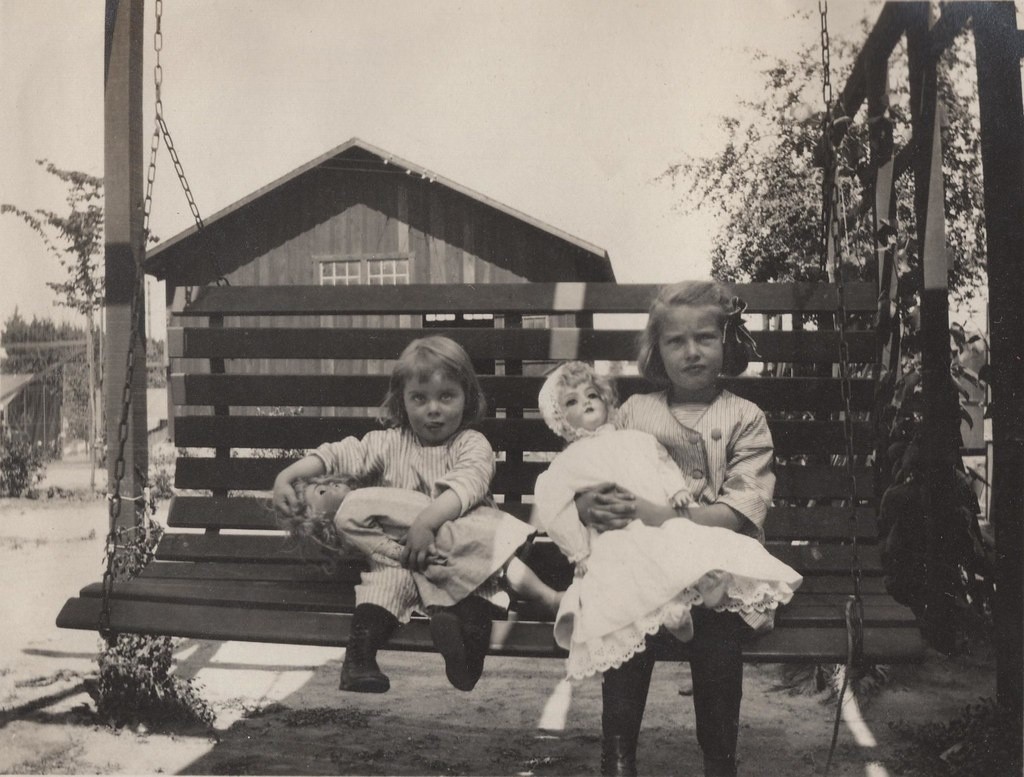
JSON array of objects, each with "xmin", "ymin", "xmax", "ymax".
[
  {"xmin": 338, "ymin": 601, "xmax": 399, "ymax": 692},
  {"xmin": 432, "ymin": 604, "xmax": 493, "ymax": 690}
]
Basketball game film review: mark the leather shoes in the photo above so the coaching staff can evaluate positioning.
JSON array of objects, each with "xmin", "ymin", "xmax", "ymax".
[{"xmin": 601, "ymin": 736, "xmax": 638, "ymax": 777}]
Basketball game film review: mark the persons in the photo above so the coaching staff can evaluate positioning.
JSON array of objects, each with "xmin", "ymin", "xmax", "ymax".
[
  {"xmin": 536, "ymin": 364, "xmax": 803, "ymax": 682},
  {"xmin": 272, "ymin": 335, "xmax": 496, "ymax": 692},
  {"xmin": 275, "ymin": 480, "xmax": 564, "ymax": 615},
  {"xmin": 576, "ymin": 279, "xmax": 777, "ymax": 777}
]
